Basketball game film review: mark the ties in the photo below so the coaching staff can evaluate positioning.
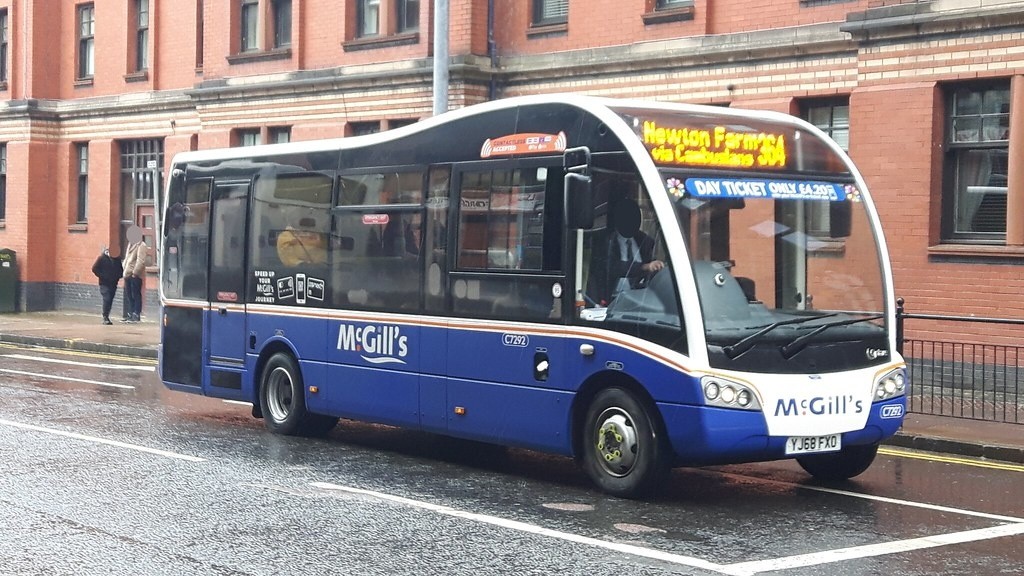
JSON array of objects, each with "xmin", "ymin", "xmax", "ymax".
[{"xmin": 626, "ymin": 239, "xmax": 636, "ymax": 273}]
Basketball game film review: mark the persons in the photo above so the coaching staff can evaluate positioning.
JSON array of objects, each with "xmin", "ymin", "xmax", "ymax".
[
  {"xmin": 276, "ymin": 200, "xmax": 340, "ymax": 279},
  {"xmin": 597, "ymin": 200, "xmax": 667, "ymax": 307},
  {"xmin": 91, "ymin": 226, "xmax": 148, "ymax": 325}
]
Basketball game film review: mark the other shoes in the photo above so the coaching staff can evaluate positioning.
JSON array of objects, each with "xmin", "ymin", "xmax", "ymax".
[
  {"xmin": 119, "ymin": 313, "xmax": 141, "ymax": 324},
  {"xmin": 102, "ymin": 317, "xmax": 113, "ymax": 325}
]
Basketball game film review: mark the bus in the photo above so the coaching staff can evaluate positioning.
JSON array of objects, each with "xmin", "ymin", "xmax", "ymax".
[{"xmin": 155, "ymin": 94, "xmax": 908, "ymax": 501}]
